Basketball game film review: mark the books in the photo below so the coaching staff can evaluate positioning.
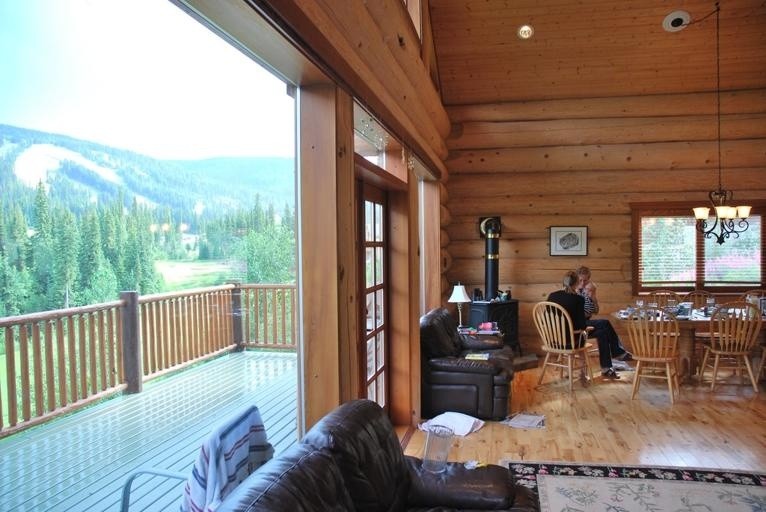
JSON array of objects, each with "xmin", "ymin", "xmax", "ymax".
[{"xmin": 464, "ymin": 352, "xmax": 489, "ymax": 361}]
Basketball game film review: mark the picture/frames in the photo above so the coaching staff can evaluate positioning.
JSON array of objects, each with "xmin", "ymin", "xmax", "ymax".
[{"xmin": 548, "ymin": 226, "xmax": 587, "ymax": 257}]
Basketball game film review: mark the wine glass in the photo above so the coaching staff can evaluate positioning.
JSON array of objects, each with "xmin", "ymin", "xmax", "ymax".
[{"xmin": 615, "ymin": 295, "xmax": 716, "ymax": 318}]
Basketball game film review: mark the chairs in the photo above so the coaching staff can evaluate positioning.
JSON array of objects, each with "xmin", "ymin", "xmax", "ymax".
[
  {"xmin": 645, "ymin": 291, "xmax": 683, "ymax": 351},
  {"xmin": 699, "ymin": 302, "xmax": 762, "ymax": 393},
  {"xmin": 627, "ymin": 306, "xmax": 681, "ymax": 404},
  {"xmin": 682, "ymin": 291, "xmax": 732, "ymax": 344},
  {"xmin": 734, "ymin": 290, "xmax": 765, "ymax": 375},
  {"xmin": 533, "ymin": 302, "xmax": 594, "ymax": 392},
  {"xmin": 560, "ymin": 327, "xmax": 600, "ymax": 379},
  {"xmin": 121, "ymin": 405, "xmax": 258, "ymax": 511}
]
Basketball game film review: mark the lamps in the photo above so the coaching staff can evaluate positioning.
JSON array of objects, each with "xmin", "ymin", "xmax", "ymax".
[
  {"xmin": 692, "ymin": 3, "xmax": 752, "ymax": 246},
  {"xmin": 448, "ymin": 282, "xmax": 472, "ymax": 327}
]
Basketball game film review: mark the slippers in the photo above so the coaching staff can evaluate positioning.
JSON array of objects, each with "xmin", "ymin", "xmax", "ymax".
[
  {"xmin": 619, "ymin": 352, "xmax": 634, "ymax": 361},
  {"xmin": 602, "ymin": 368, "xmax": 621, "ymax": 379}
]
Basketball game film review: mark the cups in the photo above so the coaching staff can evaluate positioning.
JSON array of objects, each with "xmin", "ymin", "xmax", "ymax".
[{"xmin": 421, "ymin": 424, "xmax": 454, "ymax": 475}]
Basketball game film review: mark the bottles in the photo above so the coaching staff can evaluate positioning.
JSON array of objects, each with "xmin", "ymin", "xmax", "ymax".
[{"xmin": 746, "ymin": 293, "xmax": 766, "ymax": 318}]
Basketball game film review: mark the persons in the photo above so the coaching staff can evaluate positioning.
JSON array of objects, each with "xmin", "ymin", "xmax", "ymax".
[
  {"xmin": 576, "ymin": 265, "xmax": 634, "ymax": 380},
  {"xmin": 544, "ymin": 271, "xmax": 590, "ymax": 387}
]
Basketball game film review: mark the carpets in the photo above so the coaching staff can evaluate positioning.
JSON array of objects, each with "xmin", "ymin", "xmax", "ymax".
[{"xmin": 498, "ymin": 461, "xmax": 766, "ymax": 511}]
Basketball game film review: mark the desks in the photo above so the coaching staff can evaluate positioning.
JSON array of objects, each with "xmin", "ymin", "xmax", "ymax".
[{"xmin": 611, "ymin": 309, "xmax": 766, "ymax": 375}]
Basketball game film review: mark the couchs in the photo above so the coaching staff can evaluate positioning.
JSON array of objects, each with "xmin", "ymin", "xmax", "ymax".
[
  {"xmin": 420, "ymin": 307, "xmax": 515, "ymax": 421},
  {"xmin": 213, "ymin": 399, "xmax": 538, "ymax": 510}
]
